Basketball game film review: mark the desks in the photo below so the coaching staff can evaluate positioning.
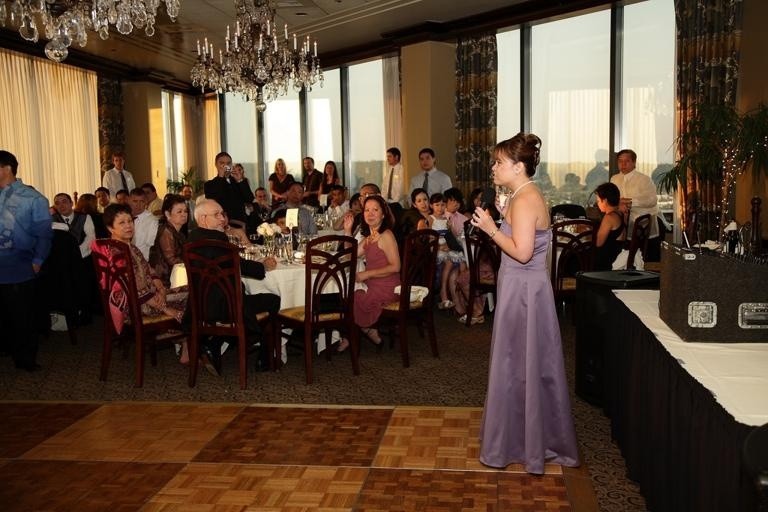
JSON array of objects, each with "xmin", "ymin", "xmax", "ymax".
[{"xmin": 611, "ymin": 285, "xmax": 768, "ymax": 511}]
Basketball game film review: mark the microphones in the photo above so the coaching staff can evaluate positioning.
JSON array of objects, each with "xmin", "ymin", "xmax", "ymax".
[{"xmin": 468, "ymin": 188, "xmax": 496, "ymax": 237}]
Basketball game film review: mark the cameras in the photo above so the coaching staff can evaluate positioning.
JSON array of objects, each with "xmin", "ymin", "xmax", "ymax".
[{"xmin": 223, "ymin": 165, "xmax": 233, "ymax": 172}]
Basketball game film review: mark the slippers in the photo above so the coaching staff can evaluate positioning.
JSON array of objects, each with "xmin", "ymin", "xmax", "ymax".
[{"xmin": 438, "ymin": 301, "xmax": 484, "ymax": 324}]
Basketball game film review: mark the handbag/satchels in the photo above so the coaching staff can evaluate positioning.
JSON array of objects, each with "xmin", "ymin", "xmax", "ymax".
[
  {"xmin": 312, "ymin": 293, "xmax": 345, "ymax": 314},
  {"xmin": 586, "ymin": 192, "xmax": 602, "ymax": 220}
]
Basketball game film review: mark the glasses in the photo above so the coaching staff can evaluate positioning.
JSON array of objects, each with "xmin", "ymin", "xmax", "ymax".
[
  {"xmin": 203, "ymin": 213, "xmax": 225, "ymax": 219},
  {"xmin": 359, "ymin": 193, "xmax": 379, "ymax": 199}
]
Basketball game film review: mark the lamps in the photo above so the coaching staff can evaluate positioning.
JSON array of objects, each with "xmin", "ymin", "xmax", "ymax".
[
  {"xmin": 189, "ymin": 0, "xmax": 324, "ymax": 113},
  {"xmin": 0, "ymin": 0, "xmax": 181, "ymax": 63}
]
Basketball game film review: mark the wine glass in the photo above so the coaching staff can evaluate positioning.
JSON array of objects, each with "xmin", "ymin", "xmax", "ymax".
[
  {"xmin": 495, "ymin": 187, "xmax": 509, "ymax": 224},
  {"xmin": 226, "ymin": 232, "xmax": 333, "ymax": 265},
  {"xmin": 553, "ymin": 212, "xmax": 590, "ymax": 234},
  {"xmin": 308, "ymin": 210, "xmax": 342, "ymax": 229}
]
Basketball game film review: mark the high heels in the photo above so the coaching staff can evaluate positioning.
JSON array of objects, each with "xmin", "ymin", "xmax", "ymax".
[
  {"xmin": 331, "ymin": 338, "xmax": 351, "ymax": 355},
  {"xmin": 366, "ymin": 328, "xmax": 389, "ymax": 350}
]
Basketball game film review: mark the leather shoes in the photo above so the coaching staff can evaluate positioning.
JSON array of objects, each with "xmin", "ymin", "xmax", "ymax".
[
  {"xmin": 256, "ymin": 359, "xmax": 284, "ymax": 372},
  {"xmin": 199, "ymin": 347, "xmax": 221, "ymax": 377}
]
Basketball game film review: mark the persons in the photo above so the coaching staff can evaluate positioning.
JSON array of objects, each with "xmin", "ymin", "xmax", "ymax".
[
  {"xmin": 268, "ymin": 158, "xmax": 323, "ymax": 250},
  {"xmin": 470, "ymin": 131, "xmax": 582, "ymax": 476},
  {"xmin": 0, "ymin": 150, "xmax": 53, "ymax": 371},
  {"xmin": 46, "ymin": 147, "xmax": 280, "ymax": 374},
  {"xmin": 591, "ymin": 183, "xmax": 627, "ymax": 271},
  {"xmin": 316, "ymin": 161, "xmax": 402, "ymax": 355},
  {"xmin": 610, "ymin": 150, "xmax": 661, "ymax": 262},
  {"xmin": 380, "ymin": 148, "xmax": 500, "ymax": 324}
]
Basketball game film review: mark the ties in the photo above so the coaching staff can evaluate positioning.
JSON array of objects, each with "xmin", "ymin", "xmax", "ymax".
[
  {"xmin": 119, "ymin": 171, "xmax": 129, "ymax": 197},
  {"xmin": 130, "ymin": 217, "xmax": 138, "ymax": 241},
  {"xmin": 388, "ymin": 168, "xmax": 393, "ymax": 199},
  {"xmin": 66, "ymin": 218, "xmax": 70, "ymax": 227},
  {"xmin": 423, "ymin": 173, "xmax": 429, "ymax": 194}
]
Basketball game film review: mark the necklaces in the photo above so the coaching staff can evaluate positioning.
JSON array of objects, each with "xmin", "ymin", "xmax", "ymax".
[{"xmin": 511, "ymin": 181, "xmax": 534, "ymax": 198}]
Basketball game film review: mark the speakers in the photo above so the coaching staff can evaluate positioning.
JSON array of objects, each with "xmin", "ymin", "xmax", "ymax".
[{"xmin": 575, "ymin": 270, "xmax": 660, "ymax": 406}]
